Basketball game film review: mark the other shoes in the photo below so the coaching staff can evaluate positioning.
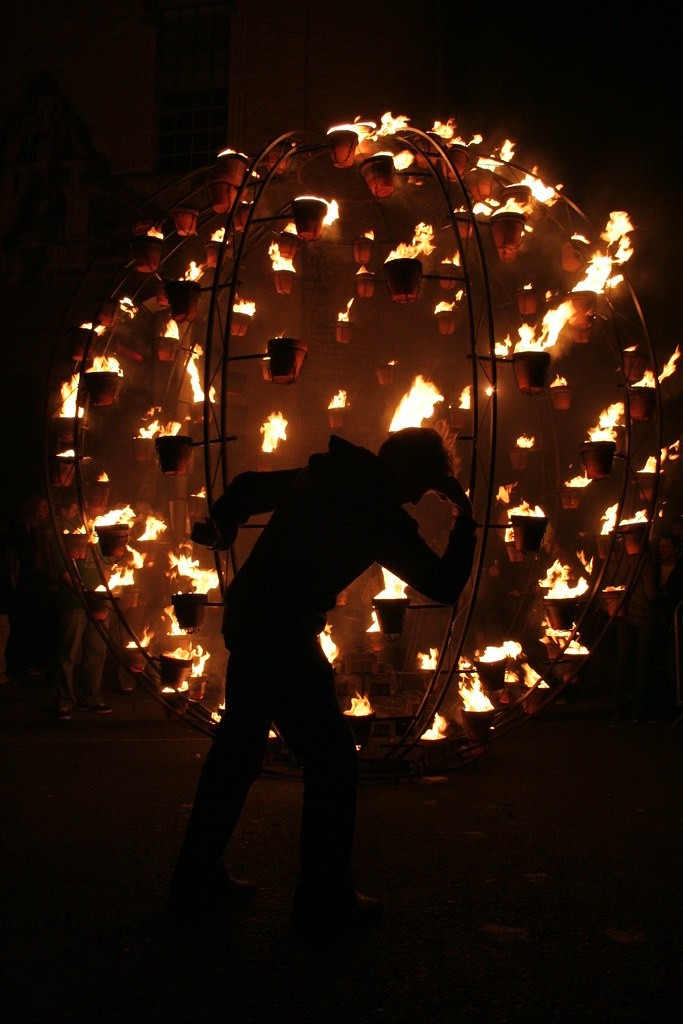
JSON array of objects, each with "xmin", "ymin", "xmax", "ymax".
[
  {"xmin": 50, "ymin": 697, "xmax": 75, "ymax": 721},
  {"xmin": 76, "ymin": 694, "xmax": 115, "ymax": 716},
  {"xmin": 284, "ymin": 890, "xmax": 386, "ymax": 923},
  {"xmin": 165, "ymin": 872, "xmax": 259, "ymax": 908}
]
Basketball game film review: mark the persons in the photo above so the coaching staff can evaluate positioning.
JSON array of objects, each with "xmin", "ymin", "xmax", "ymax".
[
  {"xmin": 0, "ymin": 518, "xmax": 114, "ymax": 720},
  {"xmin": 609, "ymin": 536, "xmax": 683, "ymax": 725},
  {"xmin": 166, "ymin": 426, "xmax": 477, "ymax": 921}
]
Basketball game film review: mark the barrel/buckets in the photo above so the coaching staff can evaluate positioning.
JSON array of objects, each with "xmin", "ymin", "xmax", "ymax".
[{"xmin": 53, "ymin": 132, "xmax": 656, "ymax": 748}]
